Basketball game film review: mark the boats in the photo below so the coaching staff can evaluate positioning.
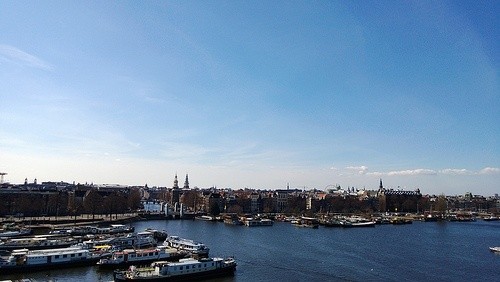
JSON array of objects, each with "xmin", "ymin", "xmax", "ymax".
[
  {"xmin": 194, "ymin": 212, "xmax": 478, "ymax": 228},
  {"xmin": 95, "ymin": 246, "xmax": 192, "ymax": 269},
  {"xmin": 483, "ymin": 215, "xmax": 500, "ymax": 220},
  {"xmin": 489, "ymin": 246, "xmax": 500, "ymax": 254},
  {"xmin": 162, "ymin": 235, "xmax": 210, "ymax": 258},
  {"xmin": 0, "ymin": 246, "xmax": 112, "ymax": 272},
  {"xmin": 113, "ymin": 254, "xmax": 239, "ymax": 282},
  {"xmin": 0, "ymin": 211, "xmax": 169, "ymax": 252}
]
